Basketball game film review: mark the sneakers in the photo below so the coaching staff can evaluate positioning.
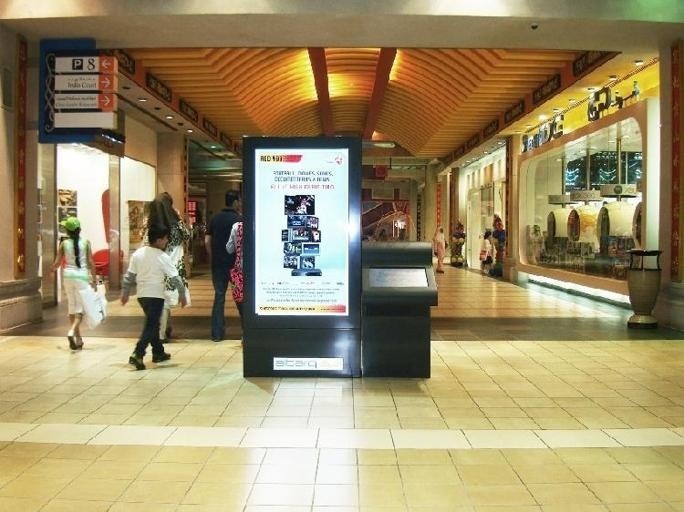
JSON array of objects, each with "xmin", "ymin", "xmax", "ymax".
[
  {"xmin": 214, "ymin": 336, "xmax": 222, "ymax": 342},
  {"xmin": 130, "ymin": 353, "xmax": 145, "ymax": 370},
  {"xmin": 160, "ymin": 339, "xmax": 168, "ymax": 343},
  {"xmin": 68, "ymin": 333, "xmax": 77, "ymax": 350},
  {"xmin": 76, "ymin": 341, "xmax": 83, "ymax": 348},
  {"xmin": 152, "ymin": 352, "xmax": 170, "ymax": 362},
  {"xmin": 165, "ymin": 328, "xmax": 171, "ymax": 338}
]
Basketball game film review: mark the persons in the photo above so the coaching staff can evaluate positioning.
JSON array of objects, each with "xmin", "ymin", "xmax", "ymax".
[
  {"xmin": 48, "ymin": 217, "xmax": 99, "ymax": 351},
  {"xmin": 154, "ymin": 191, "xmax": 183, "ymax": 222},
  {"xmin": 227, "ymin": 219, "xmax": 243, "ymax": 321},
  {"xmin": 434, "ymin": 227, "xmax": 450, "ymax": 273},
  {"xmin": 362, "ymin": 225, "xmax": 409, "ymax": 242},
  {"xmin": 205, "ymin": 189, "xmax": 240, "ymax": 343},
  {"xmin": 480, "ymin": 230, "xmax": 493, "ymax": 277},
  {"xmin": 142, "ymin": 200, "xmax": 193, "ymax": 342},
  {"xmin": 118, "ymin": 223, "xmax": 189, "ymax": 370}
]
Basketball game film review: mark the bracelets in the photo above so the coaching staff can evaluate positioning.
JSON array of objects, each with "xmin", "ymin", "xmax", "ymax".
[{"xmin": 92, "ymin": 281, "xmax": 98, "ymax": 285}]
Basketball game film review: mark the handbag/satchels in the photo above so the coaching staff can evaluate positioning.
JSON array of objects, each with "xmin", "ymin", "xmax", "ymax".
[
  {"xmin": 482, "ymin": 251, "xmax": 492, "ymax": 264},
  {"xmin": 230, "ymin": 268, "xmax": 244, "ymax": 304},
  {"xmin": 79, "ymin": 285, "xmax": 107, "ymax": 329}
]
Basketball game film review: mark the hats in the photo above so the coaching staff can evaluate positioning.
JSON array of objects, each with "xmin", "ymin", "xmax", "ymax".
[{"xmin": 61, "ymin": 218, "xmax": 80, "ymax": 231}]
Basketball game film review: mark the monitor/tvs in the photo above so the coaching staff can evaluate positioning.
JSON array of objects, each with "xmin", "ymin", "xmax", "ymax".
[
  {"xmin": 368, "ymin": 266, "xmax": 429, "ymax": 288},
  {"xmin": 280, "ymin": 194, "xmax": 322, "ymax": 270}
]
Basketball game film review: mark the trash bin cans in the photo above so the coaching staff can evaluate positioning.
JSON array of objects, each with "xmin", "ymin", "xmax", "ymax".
[{"xmin": 624, "ymin": 249, "xmax": 664, "ymax": 324}]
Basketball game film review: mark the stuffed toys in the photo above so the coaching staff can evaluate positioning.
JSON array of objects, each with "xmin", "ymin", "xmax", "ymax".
[
  {"xmin": 490, "ymin": 215, "xmax": 506, "ymax": 278},
  {"xmin": 448, "ymin": 220, "xmax": 467, "ymax": 267}
]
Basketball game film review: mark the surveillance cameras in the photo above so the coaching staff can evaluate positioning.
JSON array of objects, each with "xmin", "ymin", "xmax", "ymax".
[{"xmin": 530, "ymin": 23, "xmax": 539, "ymax": 31}]
